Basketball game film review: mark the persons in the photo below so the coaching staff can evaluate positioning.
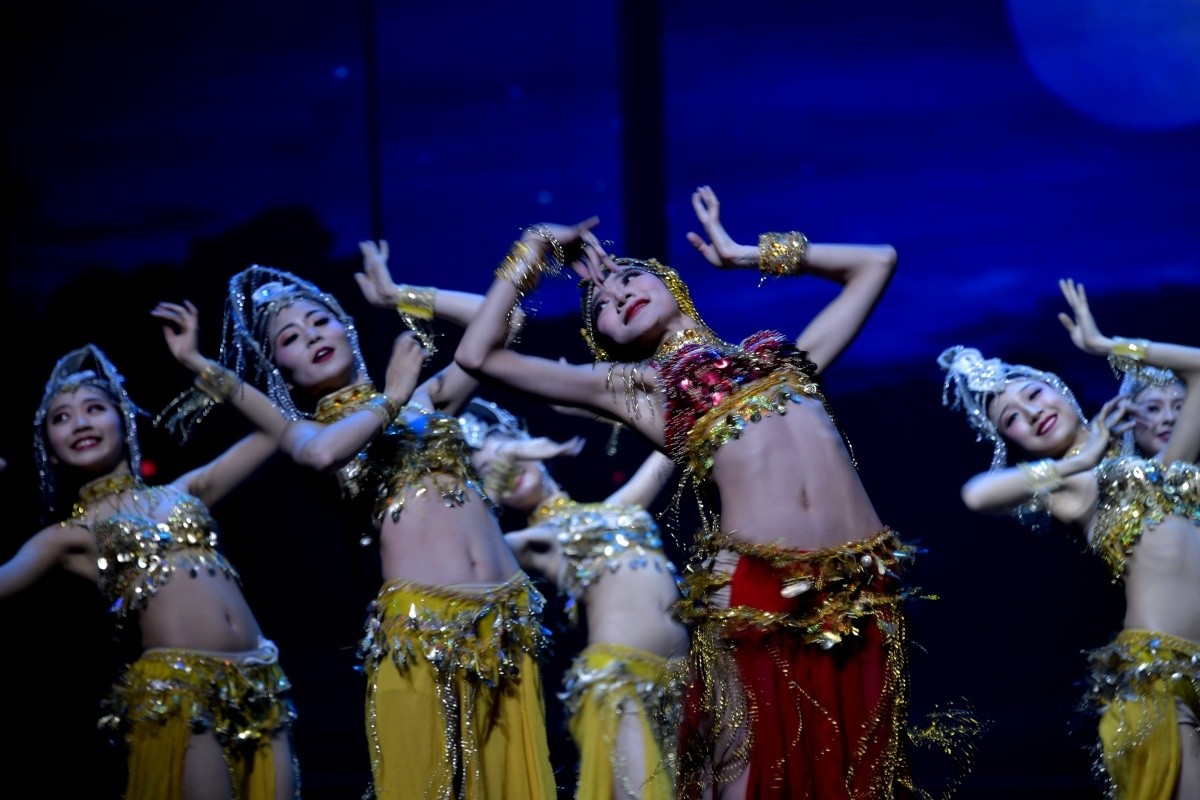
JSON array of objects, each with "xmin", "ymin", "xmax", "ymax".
[
  {"xmin": 455, "ymin": 395, "xmax": 690, "ymax": 800},
  {"xmin": 938, "ymin": 279, "xmax": 1200, "ymax": 800},
  {"xmin": 1108, "ymin": 353, "xmax": 1188, "ymax": 457},
  {"xmin": 456, "ymin": 186, "xmax": 979, "ymax": 800},
  {"xmin": 0, "ymin": 301, "xmax": 303, "ymax": 800},
  {"xmin": 219, "ymin": 240, "xmax": 558, "ymax": 800}
]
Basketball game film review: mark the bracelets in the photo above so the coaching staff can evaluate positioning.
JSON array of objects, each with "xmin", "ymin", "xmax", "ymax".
[
  {"xmin": 1109, "ymin": 336, "xmax": 1150, "ymax": 361},
  {"xmin": 1017, "ymin": 458, "xmax": 1065, "ymax": 493},
  {"xmin": 483, "ymin": 456, "xmax": 514, "ymax": 494},
  {"xmin": 759, "ymin": 232, "xmax": 808, "ymax": 278},
  {"xmin": 398, "ymin": 285, "xmax": 436, "ymax": 320},
  {"xmin": 196, "ymin": 359, "xmax": 242, "ymax": 402},
  {"xmin": 493, "ymin": 224, "xmax": 566, "ymax": 294}
]
